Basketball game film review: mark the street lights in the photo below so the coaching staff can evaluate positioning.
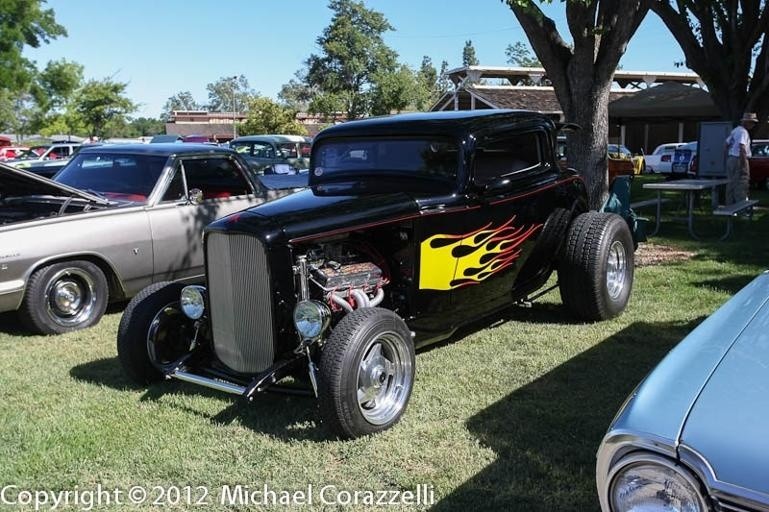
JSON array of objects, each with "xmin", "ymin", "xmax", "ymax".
[{"xmin": 231, "ymin": 76, "xmax": 240, "ymax": 140}]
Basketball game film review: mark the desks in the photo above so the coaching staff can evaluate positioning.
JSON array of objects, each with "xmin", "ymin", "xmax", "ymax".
[{"xmin": 643, "ymin": 179, "xmax": 731, "ymax": 238}]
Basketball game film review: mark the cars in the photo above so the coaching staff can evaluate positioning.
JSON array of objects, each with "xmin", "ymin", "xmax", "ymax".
[
  {"xmin": 112, "ymin": 112, "xmax": 639, "ymax": 437},
  {"xmin": 595, "ymin": 267, "xmax": 769, "ymax": 512},
  {"xmin": 1, "ymin": 141, "xmax": 309, "ymax": 339}
]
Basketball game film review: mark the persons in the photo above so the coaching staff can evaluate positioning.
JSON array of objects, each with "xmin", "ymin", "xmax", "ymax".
[
  {"xmin": 211, "ymin": 134, "xmax": 219, "ymax": 143},
  {"xmin": 725, "ymin": 112, "xmax": 758, "ymax": 216}
]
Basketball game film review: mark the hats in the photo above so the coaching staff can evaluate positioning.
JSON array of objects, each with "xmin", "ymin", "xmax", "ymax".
[{"xmin": 739, "ymin": 111, "xmax": 758, "ymax": 123}]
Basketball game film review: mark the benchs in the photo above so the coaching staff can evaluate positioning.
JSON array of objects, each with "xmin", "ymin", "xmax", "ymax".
[
  {"xmin": 714, "ymin": 199, "xmax": 759, "ymax": 240},
  {"xmin": 630, "ymin": 197, "xmax": 670, "ymax": 225}
]
